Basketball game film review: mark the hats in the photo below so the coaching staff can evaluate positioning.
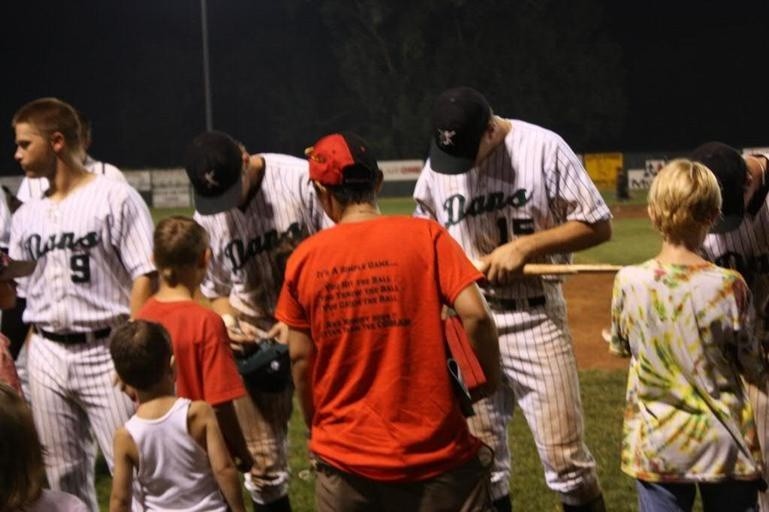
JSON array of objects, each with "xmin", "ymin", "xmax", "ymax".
[
  {"xmin": 694, "ymin": 143, "xmax": 746, "ymax": 232},
  {"xmin": 307, "ymin": 133, "xmax": 380, "ymax": 187},
  {"xmin": 183, "ymin": 133, "xmax": 242, "ymax": 215},
  {"xmin": 429, "ymin": 86, "xmax": 490, "ymax": 175}
]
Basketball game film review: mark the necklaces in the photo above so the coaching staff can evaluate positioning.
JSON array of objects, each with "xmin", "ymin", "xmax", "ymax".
[
  {"xmin": 343, "ymin": 210, "xmax": 380, "ymax": 215},
  {"xmin": 751, "ymin": 156, "xmax": 765, "ymax": 186}
]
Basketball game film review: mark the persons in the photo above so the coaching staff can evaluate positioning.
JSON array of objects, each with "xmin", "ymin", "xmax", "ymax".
[
  {"xmin": 17, "ymin": 112, "xmax": 127, "ymax": 206},
  {"xmin": 601, "ymin": 158, "xmax": 769, "ymax": 512},
  {"xmin": 186, "ymin": 129, "xmax": 336, "ymax": 511},
  {"xmin": 108, "ymin": 318, "xmax": 244, "ymax": 511},
  {"xmin": 0, "ymin": 380, "xmax": 87, "ymax": 512},
  {"xmin": 412, "ymin": 86, "xmax": 613, "ymax": 512},
  {"xmin": 125, "ymin": 215, "xmax": 254, "ymax": 473},
  {"xmin": 6, "ymin": 97, "xmax": 156, "ymax": 511},
  {"xmin": 273, "ymin": 132, "xmax": 497, "ymax": 512},
  {"xmin": 0, "ymin": 185, "xmax": 33, "ymax": 393},
  {"xmin": 693, "ymin": 141, "xmax": 769, "ymax": 512}
]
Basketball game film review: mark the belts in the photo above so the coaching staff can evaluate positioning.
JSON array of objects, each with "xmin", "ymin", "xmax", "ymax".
[
  {"xmin": 33, "ymin": 324, "xmax": 109, "ymax": 346},
  {"xmin": 485, "ymin": 294, "xmax": 546, "ymax": 309}
]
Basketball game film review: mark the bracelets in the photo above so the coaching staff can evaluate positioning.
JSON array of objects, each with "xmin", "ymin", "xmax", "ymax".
[{"xmin": 222, "ymin": 315, "xmax": 236, "ymax": 328}]
